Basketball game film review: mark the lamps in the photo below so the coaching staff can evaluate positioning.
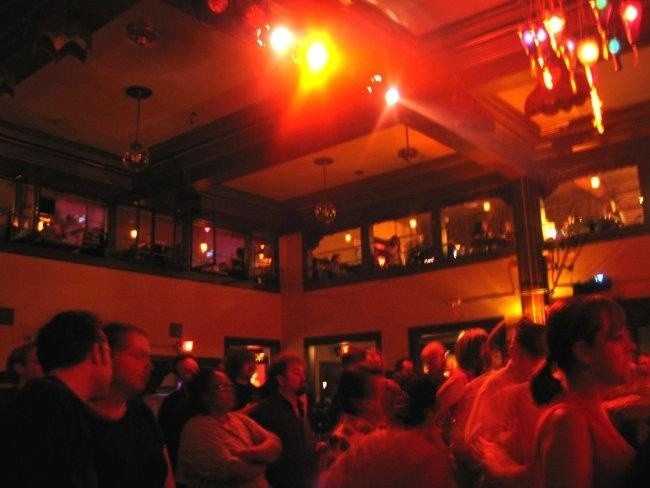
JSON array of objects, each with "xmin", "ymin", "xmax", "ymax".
[
  {"xmin": 121, "ymin": 85, "xmax": 155, "ymax": 174},
  {"xmin": 312, "ymin": 156, "xmax": 338, "ymax": 223}
]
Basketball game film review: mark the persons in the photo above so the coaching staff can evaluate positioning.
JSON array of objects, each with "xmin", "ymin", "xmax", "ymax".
[{"xmin": 1, "ymin": 200, "xmax": 650, "ymax": 488}]
